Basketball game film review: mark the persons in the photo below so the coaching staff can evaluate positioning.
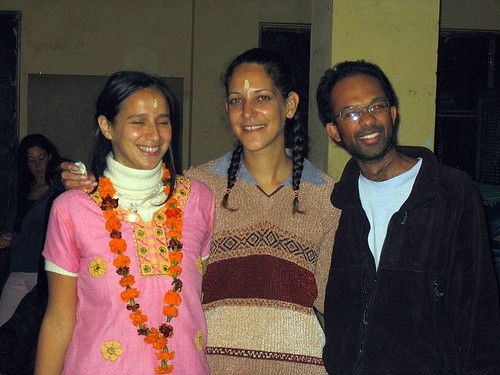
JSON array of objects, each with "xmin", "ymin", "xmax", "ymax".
[
  {"xmin": 0, "ymin": 133, "xmax": 65, "ymax": 251},
  {"xmin": 32, "ymin": 69, "xmax": 216, "ymax": 375},
  {"xmin": 56, "ymin": 48, "xmax": 346, "ymax": 375},
  {"xmin": 316, "ymin": 61, "xmax": 500, "ymax": 374}
]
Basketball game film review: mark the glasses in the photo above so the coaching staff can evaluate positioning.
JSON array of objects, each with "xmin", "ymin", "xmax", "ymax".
[{"xmin": 332, "ymin": 99, "xmax": 391, "ymax": 126}]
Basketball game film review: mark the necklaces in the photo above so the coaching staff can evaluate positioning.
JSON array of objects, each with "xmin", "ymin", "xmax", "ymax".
[{"xmin": 97, "ymin": 159, "xmax": 190, "ymax": 374}]
